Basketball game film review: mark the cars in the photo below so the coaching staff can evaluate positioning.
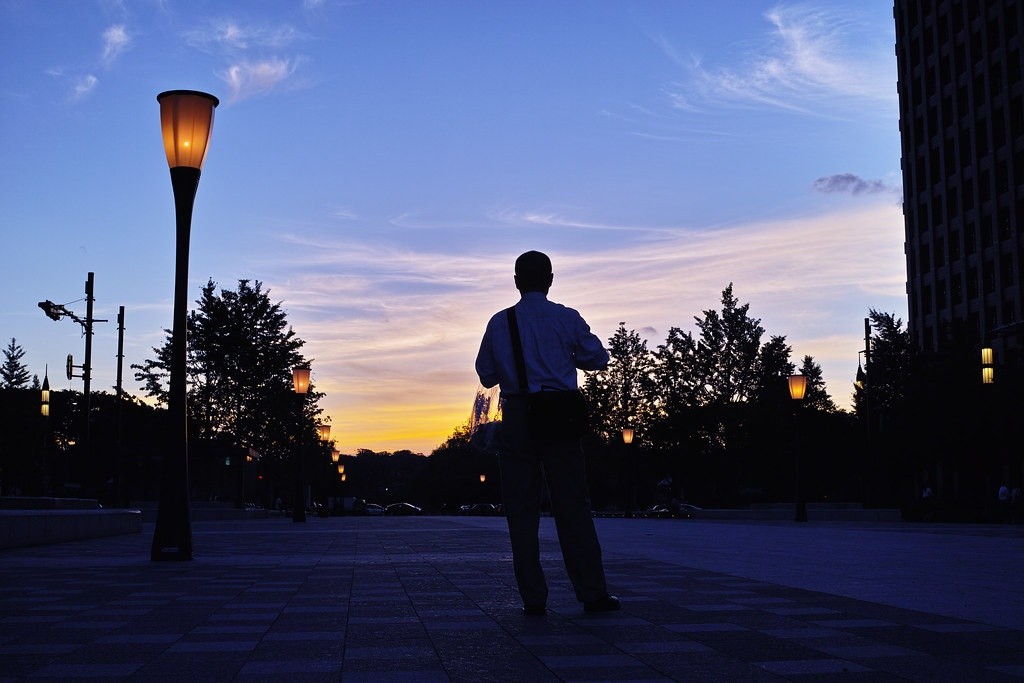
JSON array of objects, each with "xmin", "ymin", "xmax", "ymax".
[
  {"xmin": 362, "ymin": 503, "xmax": 385, "ymax": 516},
  {"xmin": 386, "ymin": 503, "xmax": 423, "ymax": 516},
  {"xmin": 467, "ymin": 504, "xmax": 496, "ymax": 516}
]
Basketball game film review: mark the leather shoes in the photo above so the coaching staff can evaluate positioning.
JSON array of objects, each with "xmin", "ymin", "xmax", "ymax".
[
  {"xmin": 523, "ymin": 599, "xmax": 547, "ymax": 614},
  {"xmin": 584, "ymin": 595, "xmax": 622, "ymax": 613}
]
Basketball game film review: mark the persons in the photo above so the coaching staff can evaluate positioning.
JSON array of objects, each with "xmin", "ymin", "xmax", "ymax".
[
  {"xmin": 475, "ymin": 251, "xmax": 622, "ymax": 617},
  {"xmin": 998, "ymin": 479, "xmax": 1023, "ymax": 524}
]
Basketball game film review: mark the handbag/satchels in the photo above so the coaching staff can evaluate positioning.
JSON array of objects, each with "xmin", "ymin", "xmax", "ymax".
[{"xmin": 527, "ymin": 385, "xmax": 588, "ymax": 446}]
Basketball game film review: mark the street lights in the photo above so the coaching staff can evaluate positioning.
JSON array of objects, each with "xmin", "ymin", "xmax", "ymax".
[
  {"xmin": 622, "ymin": 426, "xmax": 636, "ymax": 518},
  {"xmin": 318, "ymin": 423, "xmax": 330, "ymax": 517},
  {"xmin": 331, "ymin": 450, "xmax": 340, "ymax": 486},
  {"xmin": 787, "ymin": 372, "xmax": 810, "ymax": 523},
  {"xmin": 290, "ymin": 363, "xmax": 311, "ymax": 522},
  {"xmin": 148, "ymin": 88, "xmax": 221, "ymax": 564}
]
